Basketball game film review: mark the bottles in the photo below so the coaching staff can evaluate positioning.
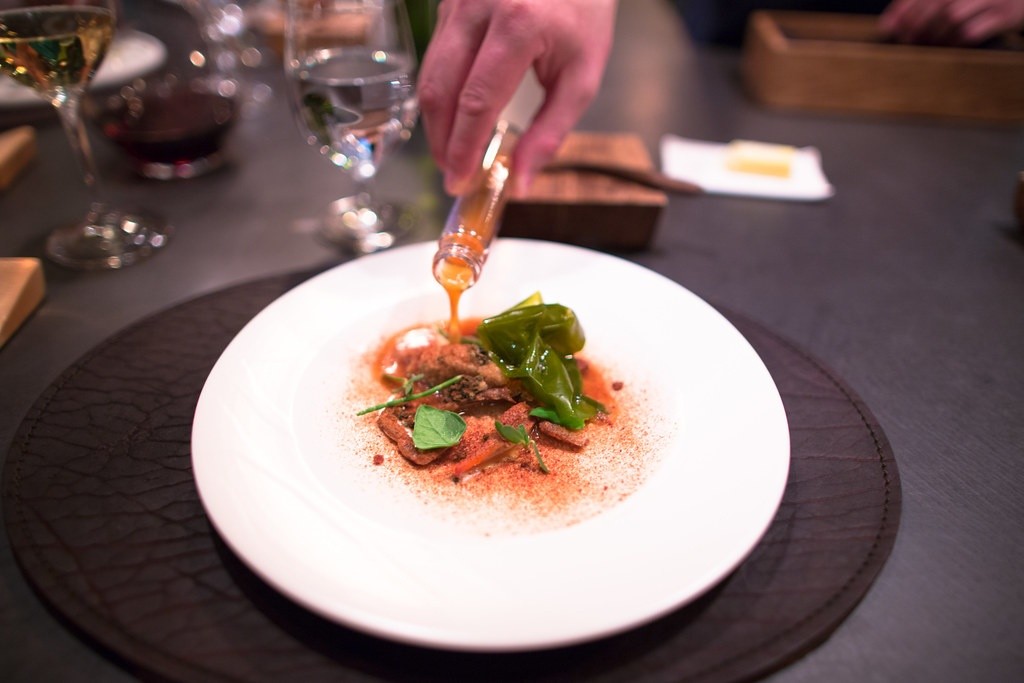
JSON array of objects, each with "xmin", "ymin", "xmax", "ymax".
[{"xmin": 432, "ymin": 119, "xmax": 524, "ymax": 291}]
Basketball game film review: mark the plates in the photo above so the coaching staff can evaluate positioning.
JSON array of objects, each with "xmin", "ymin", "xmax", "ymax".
[
  {"xmin": 190, "ymin": 236, "xmax": 789, "ymax": 652},
  {"xmin": 1, "ymin": 30, "xmax": 167, "ymax": 107}
]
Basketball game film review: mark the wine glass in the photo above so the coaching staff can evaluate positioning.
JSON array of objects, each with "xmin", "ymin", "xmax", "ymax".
[
  {"xmin": 0, "ymin": 0, "xmax": 178, "ymax": 269},
  {"xmin": 281, "ymin": 0, "xmax": 419, "ymax": 250}
]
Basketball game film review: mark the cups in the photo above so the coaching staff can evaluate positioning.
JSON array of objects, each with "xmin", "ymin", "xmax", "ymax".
[{"xmin": 80, "ymin": 2, "xmax": 240, "ymax": 179}]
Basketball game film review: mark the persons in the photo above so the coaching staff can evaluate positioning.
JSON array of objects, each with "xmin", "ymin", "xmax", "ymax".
[{"xmin": 415, "ymin": 0, "xmax": 1024, "ymax": 205}]
